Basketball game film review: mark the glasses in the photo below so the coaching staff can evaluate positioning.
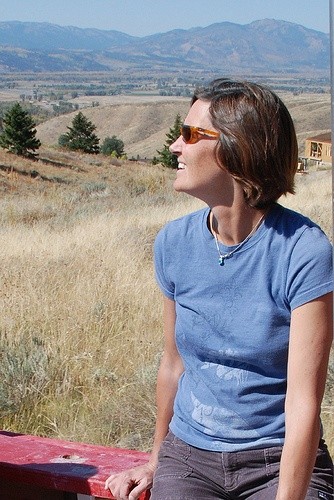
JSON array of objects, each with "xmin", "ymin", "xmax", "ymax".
[{"xmin": 180, "ymin": 125, "xmax": 220, "ymax": 144}]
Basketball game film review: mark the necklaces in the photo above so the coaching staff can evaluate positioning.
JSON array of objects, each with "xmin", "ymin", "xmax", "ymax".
[{"xmin": 212, "ymin": 208, "xmax": 270, "ymax": 265}]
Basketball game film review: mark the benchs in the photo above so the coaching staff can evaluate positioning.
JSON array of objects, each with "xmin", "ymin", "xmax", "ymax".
[{"xmin": 0, "ymin": 431, "xmax": 157, "ymax": 500}]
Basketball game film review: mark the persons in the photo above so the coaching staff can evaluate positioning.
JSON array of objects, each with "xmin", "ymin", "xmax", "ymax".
[{"xmin": 104, "ymin": 78, "xmax": 334, "ymax": 500}]
